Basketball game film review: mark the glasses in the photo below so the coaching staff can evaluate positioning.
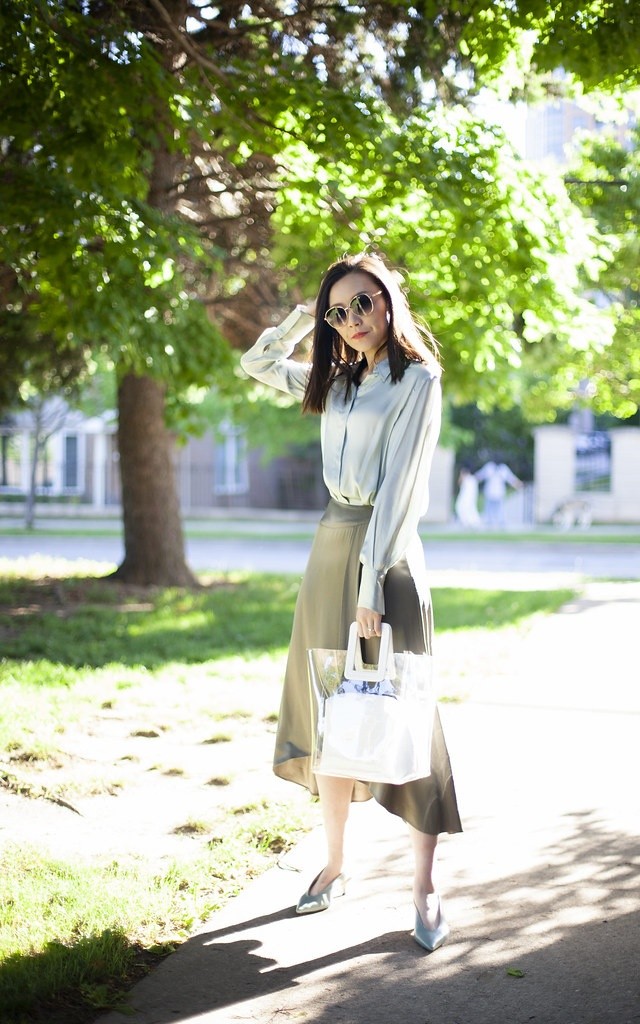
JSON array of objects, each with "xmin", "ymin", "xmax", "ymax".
[{"xmin": 324, "ymin": 290, "xmax": 382, "ymax": 329}]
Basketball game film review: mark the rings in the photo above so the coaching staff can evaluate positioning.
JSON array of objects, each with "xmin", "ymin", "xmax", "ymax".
[{"xmin": 367, "ymin": 628, "xmax": 374, "ymax": 633}]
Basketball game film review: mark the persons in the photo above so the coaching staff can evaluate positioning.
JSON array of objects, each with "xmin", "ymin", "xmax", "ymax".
[
  {"xmin": 240, "ymin": 246, "xmax": 463, "ymax": 950},
  {"xmin": 454, "ymin": 453, "xmax": 526, "ymax": 531}
]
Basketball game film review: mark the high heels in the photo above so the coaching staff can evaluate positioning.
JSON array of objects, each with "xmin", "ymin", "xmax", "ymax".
[
  {"xmin": 295, "ymin": 866, "xmax": 346, "ymax": 914},
  {"xmin": 412, "ymin": 889, "xmax": 449, "ymax": 951}
]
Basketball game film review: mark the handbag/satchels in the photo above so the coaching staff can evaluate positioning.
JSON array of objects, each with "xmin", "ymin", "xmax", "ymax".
[{"xmin": 305, "ymin": 619, "xmax": 437, "ymax": 785}]
